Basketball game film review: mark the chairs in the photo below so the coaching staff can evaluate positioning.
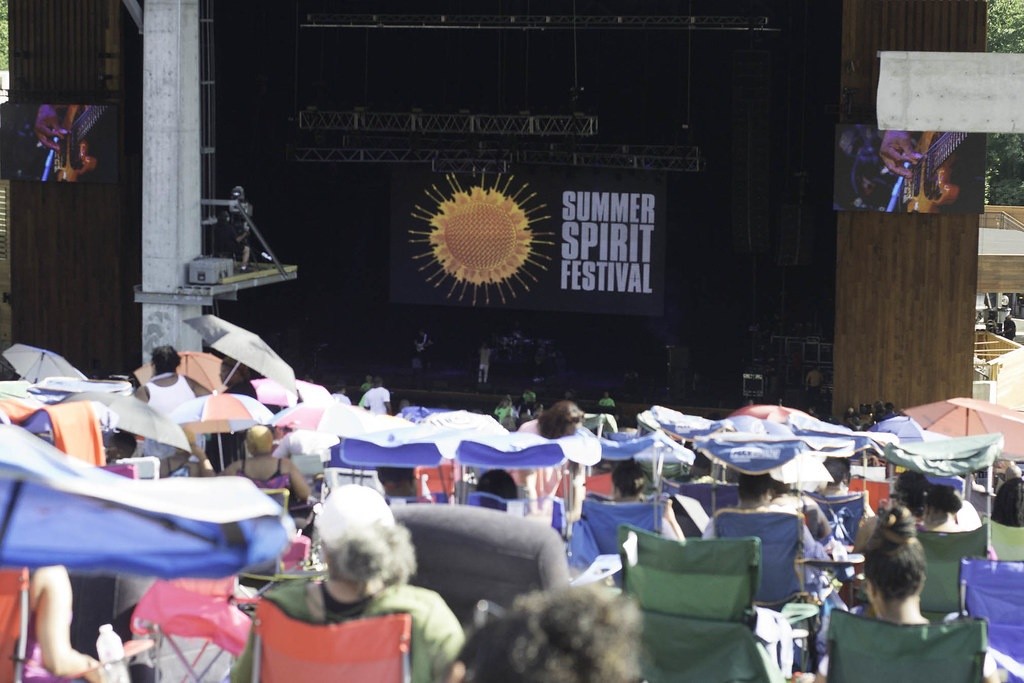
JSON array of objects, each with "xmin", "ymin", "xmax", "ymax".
[{"xmin": 0, "ymin": 464, "xmax": 1024, "ymax": 683}]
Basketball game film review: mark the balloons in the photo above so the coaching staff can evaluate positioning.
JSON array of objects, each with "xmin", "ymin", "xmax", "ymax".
[{"xmin": 250, "ymin": 378, "xmax": 330, "ymax": 406}]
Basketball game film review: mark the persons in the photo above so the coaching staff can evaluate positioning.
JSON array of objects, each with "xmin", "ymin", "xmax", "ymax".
[
  {"xmin": 414, "ymin": 329, "xmax": 433, "ymax": 368},
  {"xmin": 879, "ymin": 131, "xmax": 923, "ymax": 179},
  {"xmin": 135, "ymin": 345, "xmax": 209, "ymax": 412},
  {"xmin": 465, "ymin": 587, "xmax": 645, "ymax": 683},
  {"xmin": 359, "ymin": 375, "xmax": 410, "ymax": 416},
  {"xmin": 225, "ymin": 426, "xmax": 311, "ymax": 502},
  {"xmin": 161, "ymin": 431, "xmax": 216, "ymax": 478},
  {"xmin": 103, "ymin": 431, "xmax": 136, "ymax": 462},
  {"xmin": 0, "ymin": 567, "xmax": 102, "ymax": 683},
  {"xmin": 478, "ymin": 388, "xmax": 684, "ymax": 542},
  {"xmin": 234, "ymin": 484, "xmax": 465, "ymax": 683},
  {"xmin": 478, "ymin": 342, "xmax": 492, "ymax": 383},
  {"xmin": 206, "ymin": 358, "xmax": 257, "ymax": 469},
  {"xmin": 332, "ymin": 385, "xmax": 351, "ymax": 405},
  {"xmin": 804, "ymin": 364, "xmax": 823, "ymax": 414},
  {"xmin": 33, "ymin": 104, "xmax": 69, "ymax": 153},
  {"xmin": 663, "ymin": 428, "xmax": 1024, "ymax": 682},
  {"xmin": 842, "ymin": 400, "xmax": 894, "ymax": 430},
  {"xmin": 1000, "ymin": 315, "xmax": 1016, "ymax": 341}
]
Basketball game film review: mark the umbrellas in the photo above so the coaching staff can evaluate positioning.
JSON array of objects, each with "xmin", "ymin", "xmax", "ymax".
[
  {"xmin": 873, "ymin": 398, "xmax": 1024, "ymax": 442},
  {"xmin": 724, "ymin": 404, "xmax": 808, "ymax": 425},
  {"xmin": 176, "ymin": 393, "xmax": 412, "ymax": 468},
  {"xmin": 3, "ymin": 344, "xmax": 86, "ymax": 382},
  {"xmin": 185, "ymin": 314, "xmax": 299, "ymax": 398},
  {"xmin": 134, "ymin": 350, "xmax": 228, "ymax": 394},
  {"xmin": 61, "ymin": 391, "xmax": 189, "ymax": 452}
]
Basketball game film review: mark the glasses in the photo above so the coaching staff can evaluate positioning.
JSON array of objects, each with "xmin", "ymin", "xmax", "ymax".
[{"xmin": 564, "ymin": 409, "xmax": 585, "ymax": 425}]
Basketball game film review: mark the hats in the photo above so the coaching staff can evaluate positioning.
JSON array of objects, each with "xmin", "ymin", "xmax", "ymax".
[
  {"xmin": 246, "ymin": 425, "xmax": 275, "ymax": 456},
  {"xmin": 522, "ymin": 391, "xmax": 537, "ymax": 403},
  {"xmin": 313, "ymin": 483, "xmax": 396, "ymax": 549}
]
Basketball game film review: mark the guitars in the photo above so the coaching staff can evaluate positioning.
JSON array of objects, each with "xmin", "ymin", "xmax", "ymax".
[
  {"xmin": 902, "ymin": 131, "xmax": 971, "ymax": 214},
  {"xmin": 53, "ymin": 104, "xmax": 109, "ymax": 182}
]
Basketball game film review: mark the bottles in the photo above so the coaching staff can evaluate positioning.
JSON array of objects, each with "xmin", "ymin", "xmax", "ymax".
[{"xmin": 96, "ymin": 624, "xmax": 130, "ymax": 683}]
